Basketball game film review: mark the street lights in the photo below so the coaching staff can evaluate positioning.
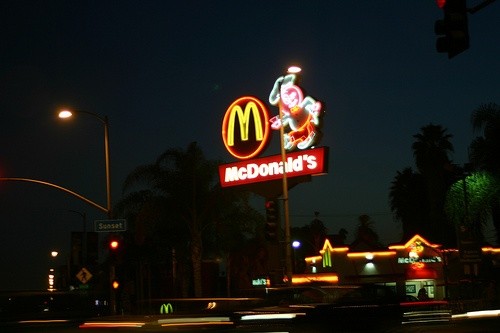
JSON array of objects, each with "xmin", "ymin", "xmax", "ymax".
[
  {"xmin": 278, "ymin": 65, "xmax": 303, "ymax": 288},
  {"xmin": 293, "ymin": 241, "xmax": 300, "ymax": 272},
  {"xmin": 51, "ymin": 251, "xmax": 70, "ymax": 279},
  {"xmin": 58, "ymin": 108, "xmax": 117, "ymax": 315}
]
